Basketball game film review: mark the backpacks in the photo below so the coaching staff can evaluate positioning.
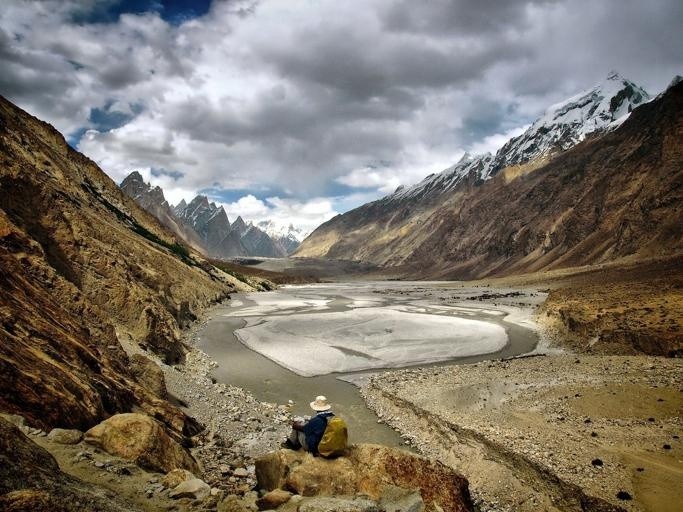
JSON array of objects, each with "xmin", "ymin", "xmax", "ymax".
[{"xmin": 317, "ymin": 413, "xmax": 349, "ymax": 457}]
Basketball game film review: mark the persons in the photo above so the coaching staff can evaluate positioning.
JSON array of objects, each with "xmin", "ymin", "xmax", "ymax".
[{"xmin": 281, "ymin": 396, "xmax": 335, "ymax": 456}]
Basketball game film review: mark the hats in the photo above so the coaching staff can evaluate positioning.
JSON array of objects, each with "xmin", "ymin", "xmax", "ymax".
[{"xmin": 309, "ymin": 396, "xmax": 331, "ymax": 411}]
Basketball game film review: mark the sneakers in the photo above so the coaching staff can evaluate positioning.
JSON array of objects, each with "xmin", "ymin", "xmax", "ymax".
[{"xmin": 282, "ymin": 438, "xmax": 302, "ymax": 451}]
